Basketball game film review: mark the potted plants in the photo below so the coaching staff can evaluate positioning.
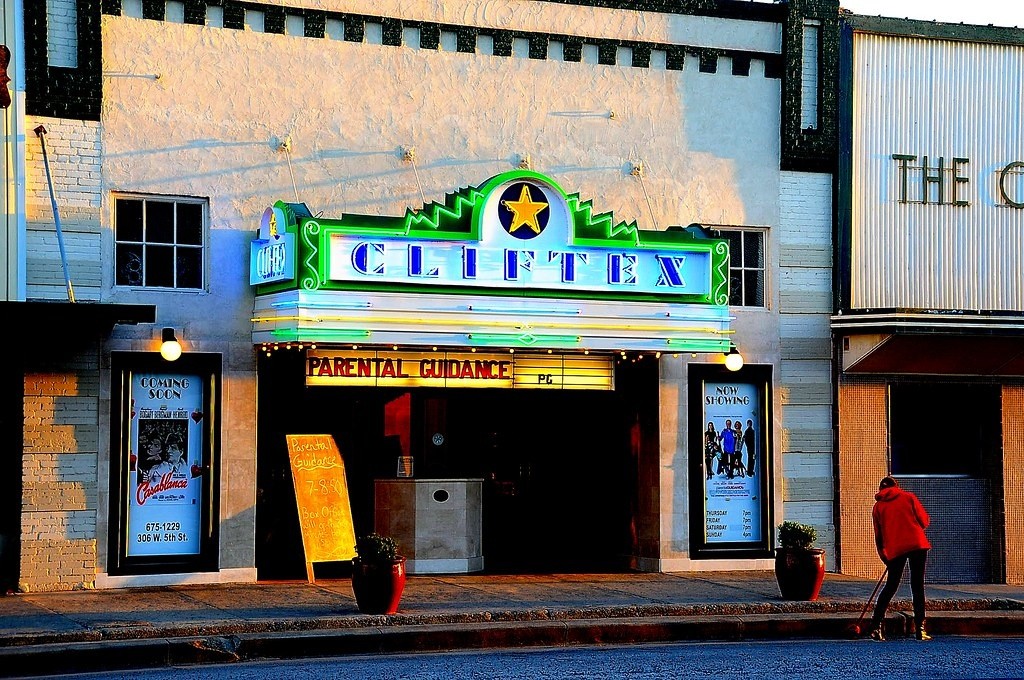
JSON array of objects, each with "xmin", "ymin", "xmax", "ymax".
[
  {"xmin": 352, "ymin": 532, "xmax": 407, "ymax": 614},
  {"xmin": 772, "ymin": 520, "xmax": 826, "ymax": 601}
]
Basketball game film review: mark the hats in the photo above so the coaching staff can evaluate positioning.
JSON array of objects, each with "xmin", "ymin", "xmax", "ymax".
[{"xmin": 880, "ymin": 477, "xmax": 898, "ymax": 487}]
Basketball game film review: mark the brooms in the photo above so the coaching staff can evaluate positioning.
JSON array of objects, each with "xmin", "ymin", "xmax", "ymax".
[{"xmin": 836, "ymin": 566, "xmax": 889, "ymax": 640}]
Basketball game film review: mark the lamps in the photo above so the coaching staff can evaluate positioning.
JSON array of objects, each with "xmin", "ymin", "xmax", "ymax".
[
  {"xmin": 160, "ymin": 328, "xmax": 181, "ymax": 360},
  {"xmin": 724, "ymin": 342, "xmax": 744, "ymax": 372}
]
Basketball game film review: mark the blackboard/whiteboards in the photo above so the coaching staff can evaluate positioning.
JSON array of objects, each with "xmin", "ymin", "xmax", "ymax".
[{"xmin": 285, "ymin": 434, "xmax": 359, "ymax": 563}]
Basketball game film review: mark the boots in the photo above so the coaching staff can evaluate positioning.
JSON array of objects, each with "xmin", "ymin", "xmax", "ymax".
[
  {"xmin": 915, "ymin": 625, "xmax": 931, "ymax": 640},
  {"xmin": 870, "ymin": 623, "xmax": 886, "ymax": 640}
]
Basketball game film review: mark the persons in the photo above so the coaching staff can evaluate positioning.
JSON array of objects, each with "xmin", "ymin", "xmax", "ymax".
[
  {"xmin": 705, "ymin": 418, "xmax": 756, "ymax": 480},
  {"xmin": 868, "ymin": 475, "xmax": 933, "ymax": 641}
]
